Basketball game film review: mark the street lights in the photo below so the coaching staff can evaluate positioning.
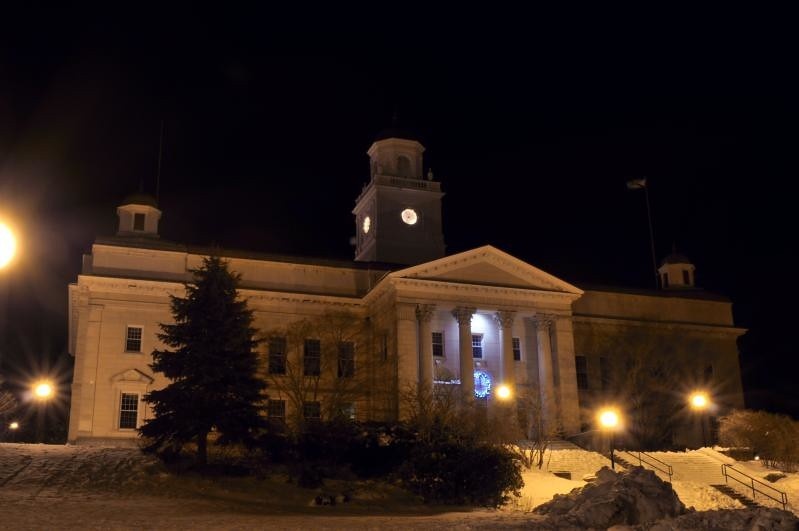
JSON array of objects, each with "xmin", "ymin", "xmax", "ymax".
[
  {"xmin": 37, "ymin": 385, "xmax": 50, "ymax": 442},
  {"xmin": 694, "ymin": 396, "xmax": 707, "ymax": 448},
  {"xmin": 498, "ymin": 387, "xmax": 510, "ymax": 446},
  {"xmin": 601, "ymin": 411, "xmax": 616, "ymax": 470}
]
